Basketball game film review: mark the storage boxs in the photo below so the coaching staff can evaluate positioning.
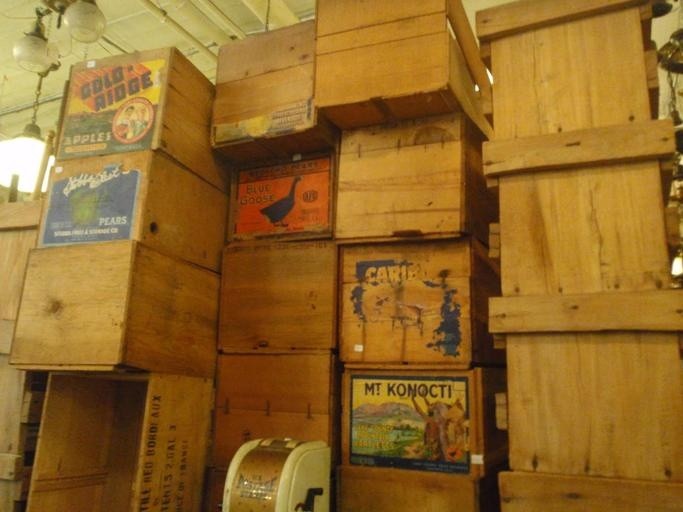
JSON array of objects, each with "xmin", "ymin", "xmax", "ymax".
[{"xmin": 0, "ymin": 0, "xmax": 683, "ymax": 512}]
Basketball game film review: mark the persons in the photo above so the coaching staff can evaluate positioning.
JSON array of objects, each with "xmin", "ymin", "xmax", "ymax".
[{"xmin": 117, "ymin": 106, "xmax": 148, "ymax": 138}]
